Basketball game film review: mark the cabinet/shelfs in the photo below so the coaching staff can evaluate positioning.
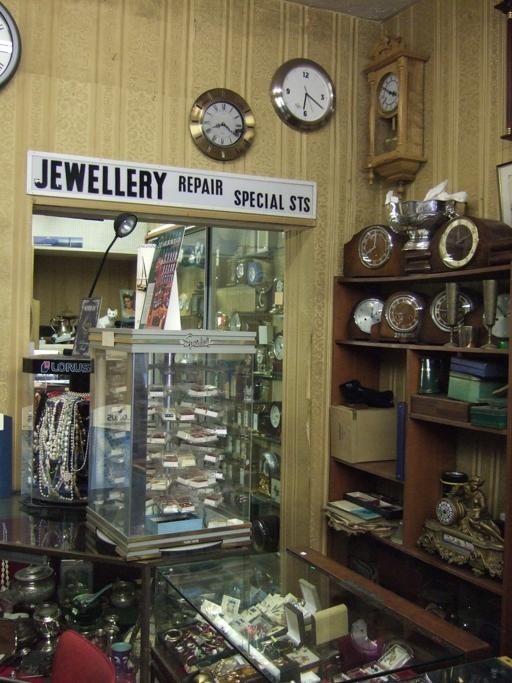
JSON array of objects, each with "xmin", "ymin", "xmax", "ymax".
[
  {"xmin": 0, "ymin": 496, "xmax": 511, "ymax": 683},
  {"xmin": 332, "ymin": 190, "xmax": 511, "ymax": 652},
  {"xmin": 143, "ymin": 226, "xmax": 288, "ymax": 557},
  {"xmin": 84, "ymin": 326, "xmax": 260, "ymax": 568}
]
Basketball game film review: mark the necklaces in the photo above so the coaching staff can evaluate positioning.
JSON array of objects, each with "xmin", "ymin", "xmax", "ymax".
[
  {"xmin": 1, "ymin": 522, "xmax": 11, "ymax": 592},
  {"xmin": 26, "ymin": 389, "xmax": 90, "ymax": 504}
]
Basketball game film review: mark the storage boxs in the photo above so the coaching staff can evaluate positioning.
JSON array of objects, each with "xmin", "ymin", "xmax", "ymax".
[{"xmin": 410, "ymin": 350, "xmax": 508, "ymax": 430}]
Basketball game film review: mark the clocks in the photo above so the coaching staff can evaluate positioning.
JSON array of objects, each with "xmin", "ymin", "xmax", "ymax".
[
  {"xmin": 429, "ymin": 212, "xmax": 510, "ymax": 274},
  {"xmin": 271, "ymin": 54, "xmax": 336, "ymax": 132},
  {"xmin": 189, "ymin": 86, "xmax": 258, "ymax": 162},
  {"xmin": 370, "ymin": 289, "xmax": 427, "ymax": 344},
  {"xmin": 0, "ymin": 5, "xmax": 24, "ymax": 85},
  {"xmin": 345, "ymin": 222, "xmax": 405, "ymax": 278},
  {"xmin": 147, "ymin": 233, "xmax": 286, "ymax": 521},
  {"xmin": 423, "ymin": 282, "xmax": 483, "ymax": 348},
  {"xmin": 415, "ymin": 470, "xmax": 510, "ymax": 582},
  {"xmin": 348, "ymin": 295, "xmax": 384, "ymax": 342},
  {"xmin": 360, "ymin": 29, "xmax": 428, "ymax": 197}
]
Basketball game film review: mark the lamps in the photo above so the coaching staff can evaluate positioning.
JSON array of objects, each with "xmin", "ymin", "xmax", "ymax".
[{"xmin": 74, "ymin": 209, "xmax": 139, "ymax": 344}]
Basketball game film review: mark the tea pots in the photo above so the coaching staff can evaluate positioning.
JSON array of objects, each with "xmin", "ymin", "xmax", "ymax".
[{"xmin": 50, "ymin": 317, "xmax": 77, "ymax": 337}]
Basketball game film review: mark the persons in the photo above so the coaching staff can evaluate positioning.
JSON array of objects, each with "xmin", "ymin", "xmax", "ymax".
[{"xmin": 121, "ymin": 293, "xmax": 136, "ymax": 316}]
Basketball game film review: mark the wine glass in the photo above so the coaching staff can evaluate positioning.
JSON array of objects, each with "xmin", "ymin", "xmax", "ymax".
[
  {"xmin": 8, "ymin": 611, "xmax": 31, "ymax": 656},
  {"xmin": 444, "ymin": 281, "xmax": 457, "ymax": 346},
  {"xmin": 482, "ymin": 280, "xmax": 497, "ymax": 348}
]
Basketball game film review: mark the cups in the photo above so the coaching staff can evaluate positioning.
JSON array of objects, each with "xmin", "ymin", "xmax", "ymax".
[
  {"xmin": 72, "ymin": 592, "xmax": 109, "ymax": 615},
  {"xmin": 462, "ymin": 324, "xmax": 479, "ymax": 348},
  {"xmin": 111, "ymin": 642, "xmax": 132, "ymax": 679},
  {"xmin": 34, "ymin": 604, "xmax": 63, "ymax": 652}
]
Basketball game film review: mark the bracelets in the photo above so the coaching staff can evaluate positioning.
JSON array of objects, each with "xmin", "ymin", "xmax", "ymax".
[
  {"xmin": 272, "ymin": 634, "xmax": 294, "ymax": 654},
  {"xmin": 162, "ymin": 620, "xmax": 256, "ymax": 682}
]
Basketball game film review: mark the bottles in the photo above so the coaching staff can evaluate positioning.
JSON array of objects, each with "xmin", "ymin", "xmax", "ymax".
[
  {"xmin": 103, "ymin": 614, "xmax": 120, "ymax": 645},
  {"xmin": 419, "ymin": 355, "xmax": 441, "ymax": 394}
]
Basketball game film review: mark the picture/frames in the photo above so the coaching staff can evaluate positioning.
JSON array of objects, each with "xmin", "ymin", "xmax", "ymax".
[{"xmin": 116, "ymin": 285, "xmax": 138, "ymax": 324}]
